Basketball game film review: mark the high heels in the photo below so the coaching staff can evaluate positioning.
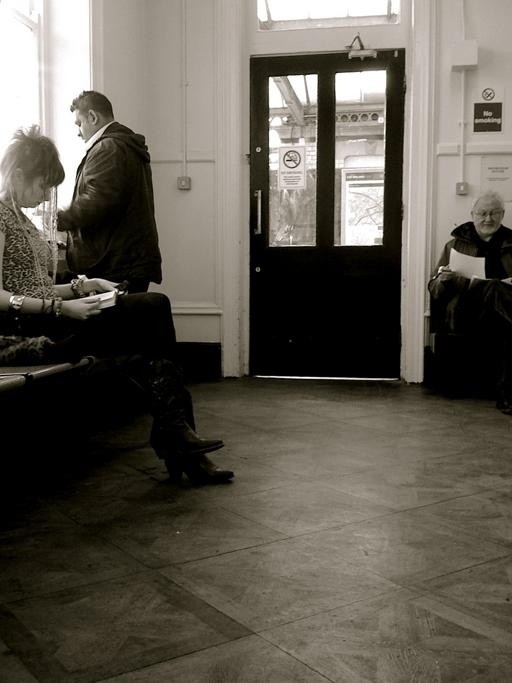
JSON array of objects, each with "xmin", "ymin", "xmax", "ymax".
[{"xmin": 152, "ymin": 419, "xmax": 234, "ymax": 483}]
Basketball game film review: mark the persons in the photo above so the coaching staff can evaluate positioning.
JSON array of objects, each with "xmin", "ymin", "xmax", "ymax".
[
  {"xmin": 39, "ymin": 89, "xmax": 162, "ymax": 434},
  {"xmin": 426, "ymin": 190, "xmax": 511, "ymax": 414},
  {"xmin": 0, "ymin": 123, "xmax": 236, "ymax": 485}
]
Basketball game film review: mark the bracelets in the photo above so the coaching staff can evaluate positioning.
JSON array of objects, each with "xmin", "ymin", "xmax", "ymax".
[
  {"xmin": 70, "ymin": 275, "xmax": 88, "ymax": 298},
  {"xmin": 41, "ymin": 299, "xmax": 56, "ymax": 315},
  {"xmin": 54, "ymin": 296, "xmax": 63, "ymax": 317},
  {"xmin": 8, "ymin": 291, "xmax": 27, "ymax": 316}
]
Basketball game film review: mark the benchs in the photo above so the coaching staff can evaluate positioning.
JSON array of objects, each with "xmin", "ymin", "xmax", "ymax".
[{"xmin": 0, "ymin": 355, "xmax": 99, "ymax": 397}]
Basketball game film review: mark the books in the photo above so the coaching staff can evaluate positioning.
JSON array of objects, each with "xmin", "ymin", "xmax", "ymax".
[{"xmin": 78, "ymin": 289, "xmax": 117, "ymax": 310}]
